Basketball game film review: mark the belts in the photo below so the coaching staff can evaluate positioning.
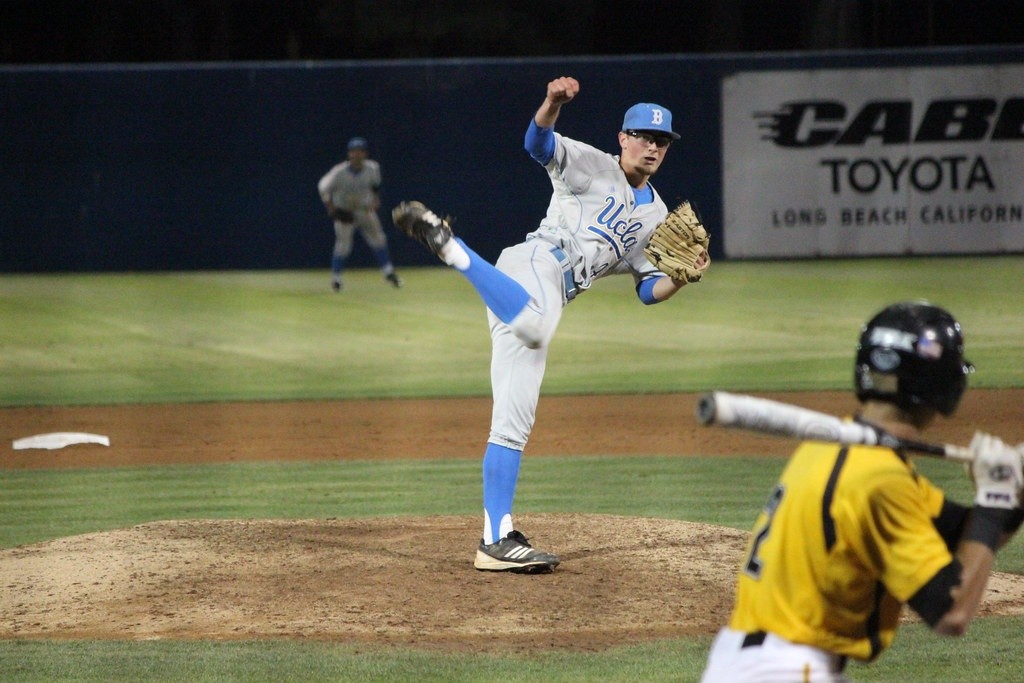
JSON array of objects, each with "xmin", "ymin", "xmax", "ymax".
[
  {"xmin": 738, "ymin": 628, "xmax": 849, "ymax": 673},
  {"xmin": 526, "ymin": 234, "xmax": 577, "ymax": 303}
]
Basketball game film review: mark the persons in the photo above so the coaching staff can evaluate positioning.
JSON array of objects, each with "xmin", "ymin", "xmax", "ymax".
[
  {"xmin": 393, "ymin": 77, "xmax": 711, "ymax": 573},
  {"xmin": 698, "ymin": 300, "xmax": 1024, "ymax": 682},
  {"xmin": 317, "ymin": 137, "xmax": 404, "ymax": 292}
]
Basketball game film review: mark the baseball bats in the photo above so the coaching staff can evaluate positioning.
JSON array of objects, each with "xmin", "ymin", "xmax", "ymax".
[{"xmin": 696, "ymin": 388, "xmax": 977, "ymax": 464}]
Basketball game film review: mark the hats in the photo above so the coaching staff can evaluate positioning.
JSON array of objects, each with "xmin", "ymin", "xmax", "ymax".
[
  {"xmin": 622, "ymin": 101, "xmax": 682, "ymax": 144},
  {"xmin": 348, "ymin": 136, "xmax": 367, "ymax": 151}
]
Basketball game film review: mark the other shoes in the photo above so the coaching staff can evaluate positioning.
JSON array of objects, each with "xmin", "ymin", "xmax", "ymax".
[
  {"xmin": 386, "ymin": 274, "xmax": 402, "ymax": 288},
  {"xmin": 332, "ymin": 281, "xmax": 342, "ymax": 291}
]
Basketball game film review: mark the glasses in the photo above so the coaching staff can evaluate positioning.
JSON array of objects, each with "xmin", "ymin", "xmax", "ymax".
[{"xmin": 622, "ymin": 129, "xmax": 674, "ymax": 147}]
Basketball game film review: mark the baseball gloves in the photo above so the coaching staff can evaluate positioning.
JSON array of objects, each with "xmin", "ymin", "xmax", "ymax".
[{"xmin": 642, "ymin": 199, "xmax": 713, "ymax": 285}]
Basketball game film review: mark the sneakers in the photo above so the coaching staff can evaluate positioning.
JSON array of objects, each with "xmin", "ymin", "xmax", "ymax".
[
  {"xmin": 474, "ymin": 529, "xmax": 560, "ymax": 575},
  {"xmin": 391, "ymin": 198, "xmax": 456, "ymax": 256}
]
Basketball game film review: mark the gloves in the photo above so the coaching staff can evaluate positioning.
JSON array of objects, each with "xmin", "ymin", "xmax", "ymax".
[{"xmin": 965, "ymin": 428, "xmax": 1024, "ymax": 535}]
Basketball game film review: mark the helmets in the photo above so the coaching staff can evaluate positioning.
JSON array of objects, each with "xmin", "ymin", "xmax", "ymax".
[{"xmin": 854, "ymin": 299, "xmax": 969, "ymax": 421}]
